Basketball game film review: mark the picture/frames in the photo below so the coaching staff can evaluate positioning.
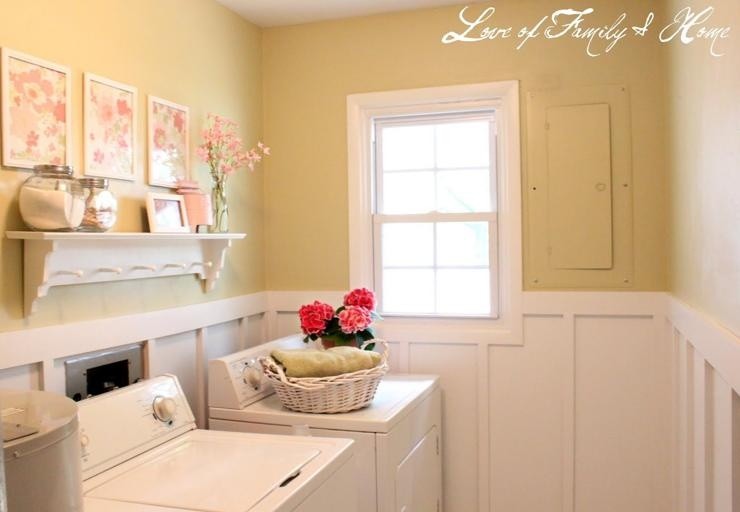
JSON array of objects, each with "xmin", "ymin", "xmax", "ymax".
[
  {"xmin": 147, "ymin": 95, "xmax": 189, "ymax": 188},
  {"xmin": 1, "ymin": 47, "xmax": 73, "ymax": 170},
  {"xmin": 145, "ymin": 192, "xmax": 190, "ymax": 233},
  {"xmin": 82, "ymin": 73, "xmax": 137, "ymax": 181},
  {"xmin": 195, "ymin": 112, "xmax": 270, "ymax": 182}
]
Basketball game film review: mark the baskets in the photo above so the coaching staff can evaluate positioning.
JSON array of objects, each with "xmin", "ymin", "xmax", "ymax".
[{"xmin": 259, "ymin": 338, "xmax": 390, "ymax": 413}]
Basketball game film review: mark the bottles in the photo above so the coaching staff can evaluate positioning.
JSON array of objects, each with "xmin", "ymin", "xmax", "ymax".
[{"xmin": 18, "ymin": 164, "xmax": 118, "ymax": 232}]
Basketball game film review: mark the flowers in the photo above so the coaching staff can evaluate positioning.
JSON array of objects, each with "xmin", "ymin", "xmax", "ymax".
[{"xmin": 299, "ymin": 288, "xmax": 384, "ymax": 350}]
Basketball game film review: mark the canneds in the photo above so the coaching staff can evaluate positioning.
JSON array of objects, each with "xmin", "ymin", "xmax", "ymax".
[
  {"xmin": 78, "ymin": 178, "xmax": 117, "ymax": 233},
  {"xmin": 17, "ymin": 163, "xmax": 86, "ymax": 232}
]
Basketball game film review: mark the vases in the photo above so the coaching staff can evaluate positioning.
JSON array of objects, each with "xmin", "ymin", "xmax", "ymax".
[
  {"xmin": 321, "ymin": 336, "xmax": 362, "ymax": 350},
  {"xmin": 209, "ymin": 172, "xmax": 228, "ymax": 233}
]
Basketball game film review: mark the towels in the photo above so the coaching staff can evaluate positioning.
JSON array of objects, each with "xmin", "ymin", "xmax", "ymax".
[{"xmin": 270, "ymin": 346, "xmax": 381, "ymax": 377}]
